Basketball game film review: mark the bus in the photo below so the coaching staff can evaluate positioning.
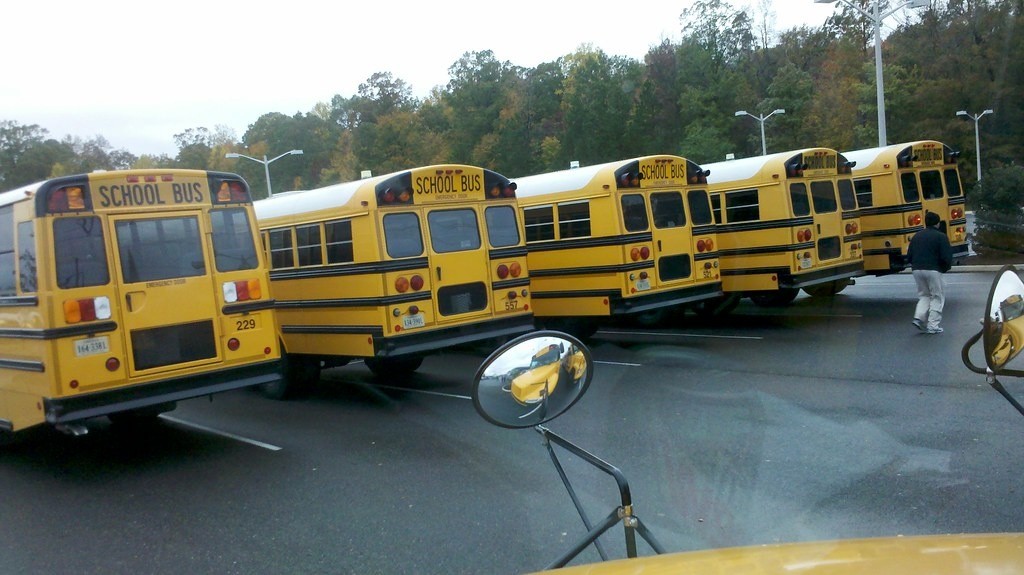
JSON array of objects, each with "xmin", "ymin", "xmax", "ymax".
[
  {"xmin": 0, "ymin": 168, "xmax": 278, "ymax": 444},
  {"xmin": 692, "ymin": 146, "xmax": 868, "ymax": 312},
  {"xmin": 839, "ymin": 139, "xmax": 969, "ymax": 276},
  {"xmin": 498, "ymin": 152, "xmax": 722, "ymax": 336},
  {"xmin": 238, "ymin": 161, "xmax": 531, "ymax": 373}
]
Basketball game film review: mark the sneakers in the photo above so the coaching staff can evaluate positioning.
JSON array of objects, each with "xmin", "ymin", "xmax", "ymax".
[
  {"xmin": 912, "ymin": 318, "xmax": 927, "ymax": 331},
  {"xmin": 925, "ymin": 325, "xmax": 943, "ymax": 333}
]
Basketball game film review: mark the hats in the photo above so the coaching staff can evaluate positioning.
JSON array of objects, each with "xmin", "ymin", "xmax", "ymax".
[{"xmin": 925, "ymin": 212, "xmax": 941, "ymax": 226}]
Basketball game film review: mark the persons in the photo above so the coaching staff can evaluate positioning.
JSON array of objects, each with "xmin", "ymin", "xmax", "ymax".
[{"xmin": 906, "ymin": 212, "xmax": 955, "ymax": 334}]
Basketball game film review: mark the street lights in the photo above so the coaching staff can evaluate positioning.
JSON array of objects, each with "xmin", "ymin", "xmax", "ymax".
[
  {"xmin": 957, "ymin": 109, "xmax": 996, "ymax": 185},
  {"xmin": 223, "ymin": 149, "xmax": 304, "ymax": 197},
  {"xmin": 734, "ymin": 108, "xmax": 786, "ymax": 154}
]
